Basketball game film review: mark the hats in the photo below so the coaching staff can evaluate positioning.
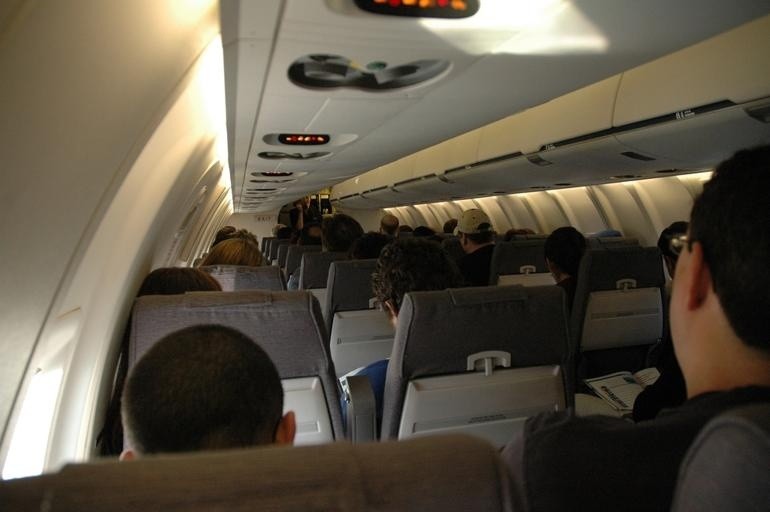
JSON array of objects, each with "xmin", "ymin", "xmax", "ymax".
[{"xmin": 453, "ymin": 209, "xmax": 490, "ymax": 236}]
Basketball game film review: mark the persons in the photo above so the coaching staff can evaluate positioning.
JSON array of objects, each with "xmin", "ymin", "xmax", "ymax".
[
  {"xmin": 94, "ymin": 264, "xmax": 224, "ymax": 452},
  {"xmin": 573, "ymin": 362, "xmax": 686, "ymax": 423},
  {"xmin": 114, "ymin": 319, "xmax": 297, "ymax": 460},
  {"xmin": 502, "ymin": 140, "xmax": 768, "ymax": 510},
  {"xmin": 198, "ymin": 192, "xmax": 693, "ymax": 293},
  {"xmin": 335, "ymin": 235, "xmax": 465, "ymax": 437}
]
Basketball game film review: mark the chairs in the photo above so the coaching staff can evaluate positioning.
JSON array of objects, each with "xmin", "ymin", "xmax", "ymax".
[{"xmin": 0, "ymin": 226, "xmax": 770, "ymax": 512}]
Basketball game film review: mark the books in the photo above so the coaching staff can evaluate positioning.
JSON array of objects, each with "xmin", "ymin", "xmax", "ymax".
[{"xmin": 579, "ymin": 367, "xmax": 660, "ymax": 413}]
